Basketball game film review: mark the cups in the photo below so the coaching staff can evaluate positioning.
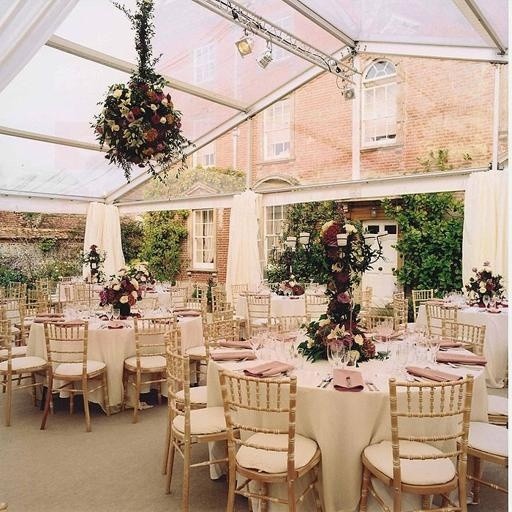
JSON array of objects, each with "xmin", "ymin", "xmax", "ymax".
[{"xmin": 327, "ymin": 340, "xmax": 351, "ymax": 370}]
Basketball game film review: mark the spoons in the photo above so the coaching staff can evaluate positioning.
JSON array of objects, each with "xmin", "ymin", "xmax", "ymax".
[{"xmin": 316, "ymin": 374, "xmax": 329, "ymax": 388}]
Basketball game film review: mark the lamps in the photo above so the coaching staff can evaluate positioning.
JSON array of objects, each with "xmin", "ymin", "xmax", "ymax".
[
  {"xmin": 256, "ymin": 48, "xmax": 273, "ymax": 69},
  {"xmin": 341, "ymin": 84, "xmax": 355, "ymax": 100},
  {"xmin": 235, "ymin": 34, "xmax": 255, "ymax": 58}
]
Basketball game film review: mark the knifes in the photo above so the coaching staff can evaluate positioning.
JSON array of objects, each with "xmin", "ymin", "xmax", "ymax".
[{"xmin": 323, "ymin": 377, "xmax": 333, "ymax": 388}]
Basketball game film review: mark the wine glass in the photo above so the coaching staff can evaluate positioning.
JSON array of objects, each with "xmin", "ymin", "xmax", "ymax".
[
  {"xmin": 441, "ymin": 288, "xmax": 503, "ymax": 313},
  {"xmin": 372, "ymin": 320, "xmax": 440, "ymax": 367},
  {"xmin": 247, "ymin": 320, "xmax": 300, "ymax": 363},
  {"xmin": 135, "ymin": 293, "xmax": 177, "ymax": 317},
  {"xmin": 64, "ymin": 302, "xmax": 121, "ymax": 327}
]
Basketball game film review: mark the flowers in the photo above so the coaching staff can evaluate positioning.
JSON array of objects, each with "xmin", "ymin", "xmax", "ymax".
[
  {"xmin": 89, "ymin": 2, "xmax": 196, "ymax": 187},
  {"xmin": 465, "ymin": 262, "xmax": 506, "ymax": 307},
  {"xmin": 264, "ymin": 202, "xmax": 390, "ymax": 363},
  {"xmin": 118, "ymin": 265, "xmax": 156, "ymax": 291}
]
